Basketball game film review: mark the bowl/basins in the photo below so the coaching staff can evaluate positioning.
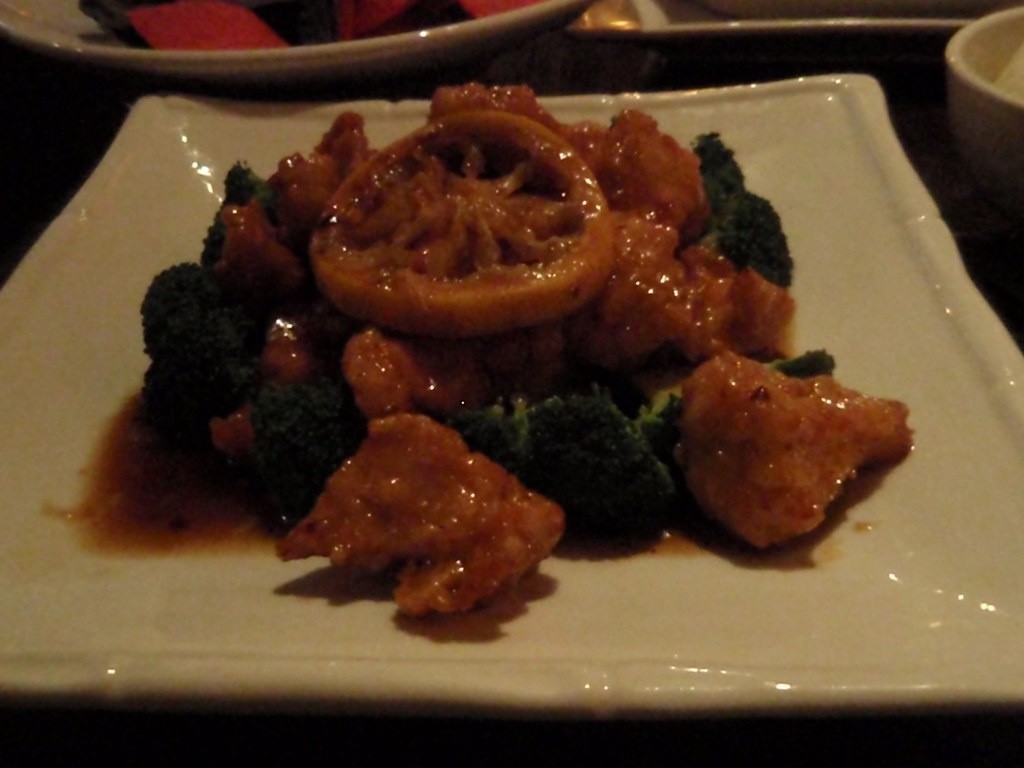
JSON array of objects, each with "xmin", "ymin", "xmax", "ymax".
[{"xmin": 944, "ymin": 4, "xmax": 1023, "ymax": 221}]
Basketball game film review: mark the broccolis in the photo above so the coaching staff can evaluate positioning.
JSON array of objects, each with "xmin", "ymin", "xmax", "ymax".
[{"xmin": 140, "ymin": 128, "xmax": 839, "ymax": 540}]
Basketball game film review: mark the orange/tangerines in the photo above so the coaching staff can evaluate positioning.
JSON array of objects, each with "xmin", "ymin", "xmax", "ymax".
[{"xmin": 308, "ymin": 106, "xmax": 607, "ymax": 334}]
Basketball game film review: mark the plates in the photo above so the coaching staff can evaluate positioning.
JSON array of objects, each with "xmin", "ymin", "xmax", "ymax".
[
  {"xmin": 567, "ymin": 0, "xmax": 1002, "ymax": 48},
  {"xmin": 2, "ymin": 0, "xmax": 600, "ymax": 102},
  {"xmin": 0, "ymin": 88, "xmax": 1024, "ymax": 747}
]
